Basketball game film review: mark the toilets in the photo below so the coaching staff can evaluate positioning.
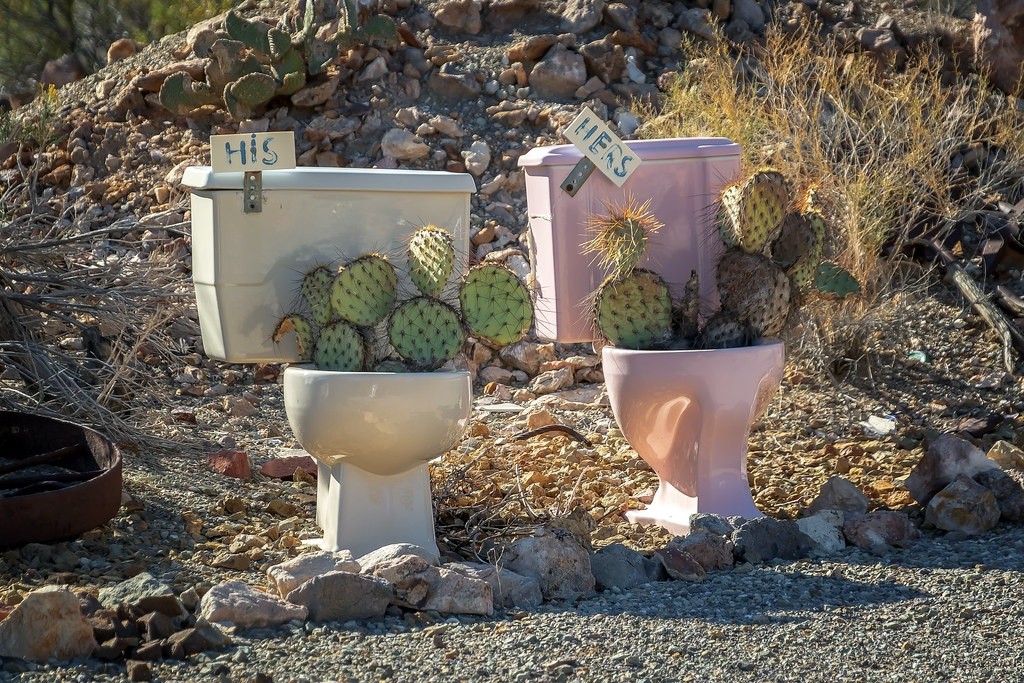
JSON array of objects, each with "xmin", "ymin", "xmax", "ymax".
[
  {"xmin": 180, "ymin": 170, "xmax": 482, "ymax": 565},
  {"xmin": 517, "ymin": 135, "xmax": 789, "ymax": 537}
]
